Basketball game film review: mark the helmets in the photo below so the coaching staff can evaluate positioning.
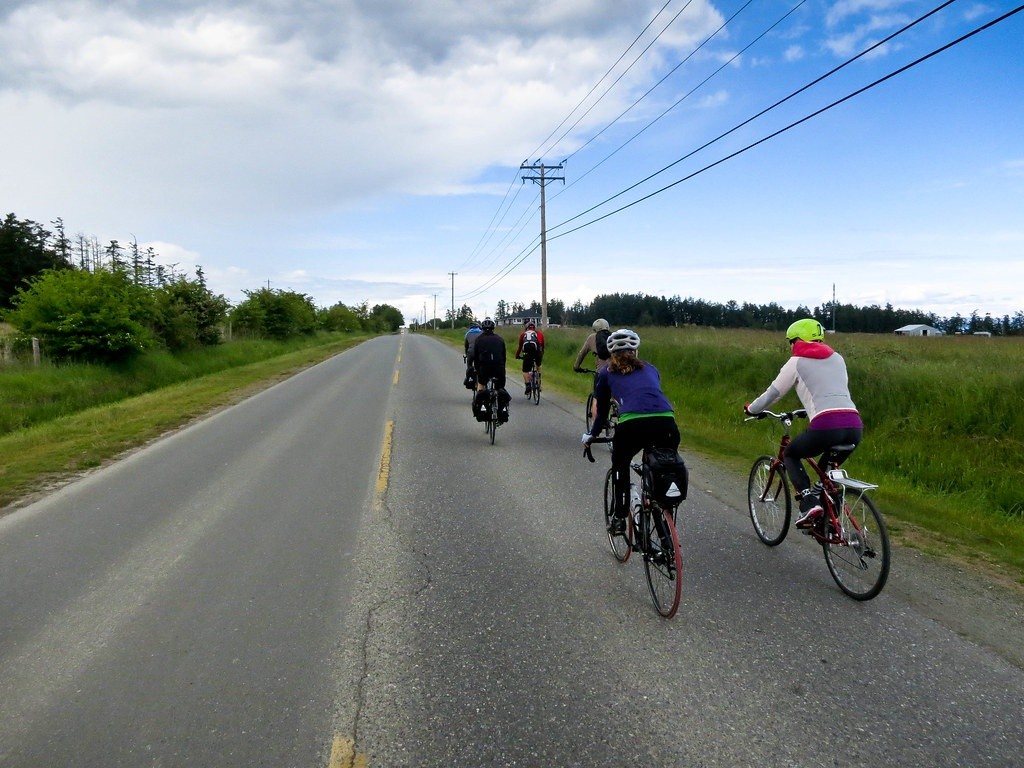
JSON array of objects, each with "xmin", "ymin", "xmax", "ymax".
[
  {"xmin": 592, "ymin": 319, "xmax": 610, "ymax": 332},
  {"xmin": 786, "ymin": 318, "xmax": 824, "ymax": 341},
  {"xmin": 525, "ymin": 321, "xmax": 535, "ymax": 329},
  {"xmin": 607, "ymin": 329, "xmax": 640, "ymax": 353},
  {"xmin": 470, "ymin": 322, "xmax": 479, "ymax": 328},
  {"xmin": 482, "ymin": 320, "xmax": 494, "ymax": 330}
]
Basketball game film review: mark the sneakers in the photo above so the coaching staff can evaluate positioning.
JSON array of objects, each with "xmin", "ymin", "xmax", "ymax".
[
  {"xmin": 808, "ymin": 515, "xmax": 840, "ymax": 534},
  {"xmin": 795, "ymin": 497, "xmax": 823, "ymax": 529},
  {"xmin": 607, "ymin": 520, "xmax": 625, "ymax": 534},
  {"xmin": 661, "ymin": 537, "xmax": 673, "ymax": 549}
]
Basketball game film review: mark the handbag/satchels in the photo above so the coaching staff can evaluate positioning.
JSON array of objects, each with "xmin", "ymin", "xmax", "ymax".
[
  {"xmin": 641, "ymin": 444, "xmax": 688, "ymax": 500},
  {"xmin": 473, "ymin": 388, "xmax": 495, "ymax": 422},
  {"xmin": 498, "ymin": 388, "xmax": 512, "ymax": 422}
]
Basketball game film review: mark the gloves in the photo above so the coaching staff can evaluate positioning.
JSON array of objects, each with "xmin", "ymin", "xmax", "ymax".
[{"xmin": 743, "ymin": 403, "xmax": 751, "ymax": 414}]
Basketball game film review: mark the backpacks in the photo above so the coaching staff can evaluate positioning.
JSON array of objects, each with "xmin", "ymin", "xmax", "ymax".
[
  {"xmin": 596, "ymin": 331, "xmax": 613, "ymax": 360},
  {"xmin": 522, "ymin": 330, "xmax": 540, "ymax": 353}
]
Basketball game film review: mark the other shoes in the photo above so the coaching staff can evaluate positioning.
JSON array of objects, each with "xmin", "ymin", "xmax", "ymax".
[
  {"xmin": 525, "ymin": 386, "xmax": 531, "ymax": 395},
  {"xmin": 536, "ymin": 380, "xmax": 541, "ymax": 384}
]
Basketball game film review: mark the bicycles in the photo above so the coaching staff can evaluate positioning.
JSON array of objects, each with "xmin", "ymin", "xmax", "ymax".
[
  {"xmin": 581, "ymin": 438, "xmax": 683, "ymax": 619},
  {"xmin": 517, "ymin": 356, "xmax": 541, "ymax": 406},
  {"xmin": 576, "ymin": 367, "xmax": 621, "ymax": 452},
  {"xmin": 463, "ymin": 354, "xmax": 498, "ymax": 446},
  {"xmin": 743, "ymin": 409, "xmax": 891, "ymax": 602}
]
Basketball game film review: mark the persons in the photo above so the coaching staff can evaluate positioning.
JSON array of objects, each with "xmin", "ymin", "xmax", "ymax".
[
  {"xmin": 743, "ymin": 318, "xmax": 863, "ymax": 530},
  {"xmin": 581, "ymin": 329, "xmax": 680, "ymax": 552},
  {"xmin": 514, "ymin": 322, "xmax": 544, "ymax": 395},
  {"xmin": 573, "ymin": 319, "xmax": 613, "ymax": 423},
  {"xmin": 473, "ymin": 319, "xmax": 507, "ymax": 393},
  {"xmin": 464, "ymin": 322, "xmax": 482, "ymax": 378}
]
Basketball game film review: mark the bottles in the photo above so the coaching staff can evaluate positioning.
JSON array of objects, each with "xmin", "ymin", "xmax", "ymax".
[
  {"xmin": 811, "ymin": 481, "xmax": 823, "ymax": 499},
  {"xmin": 629, "ymin": 485, "xmax": 643, "ymax": 525}
]
